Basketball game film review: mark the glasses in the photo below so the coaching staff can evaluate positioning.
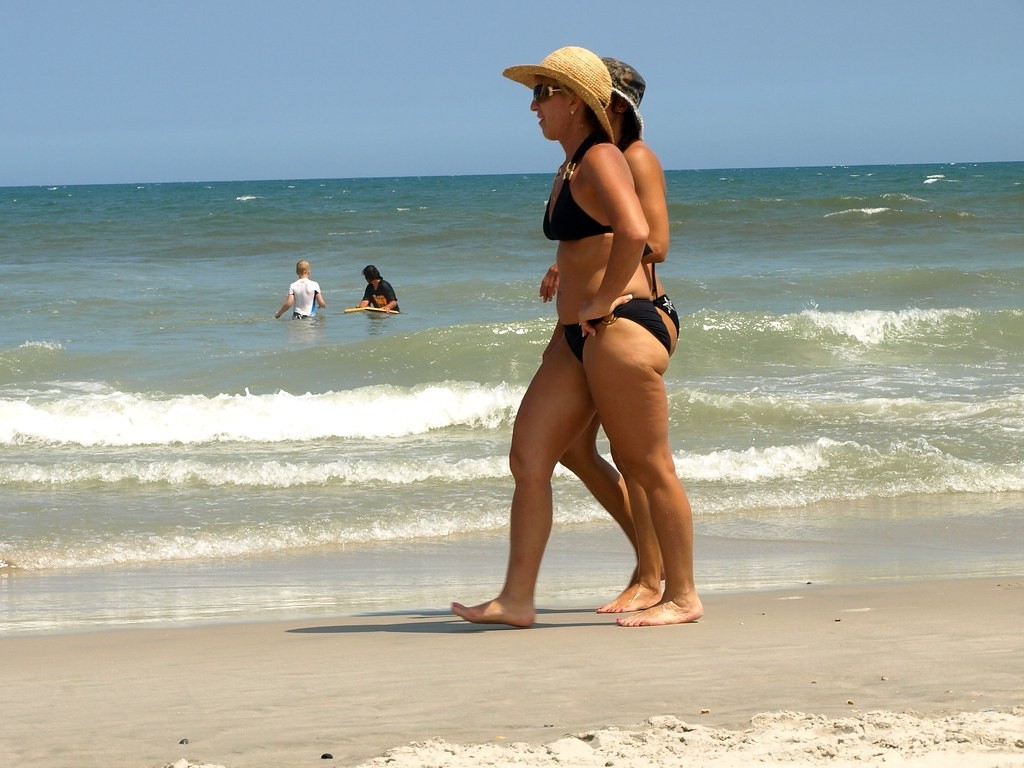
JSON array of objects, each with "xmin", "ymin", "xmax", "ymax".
[{"xmin": 533, "ymin": 84, "xmax": 563, "ymax": 101}]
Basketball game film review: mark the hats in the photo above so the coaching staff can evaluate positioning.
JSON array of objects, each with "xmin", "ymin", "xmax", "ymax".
[
  {"xmin": 502, "ymin": 45, "xmax": 615, "ymax": 143},
  {"xmin": 599, "ymin": 57, "xmax": 646, "ymax": 141}
]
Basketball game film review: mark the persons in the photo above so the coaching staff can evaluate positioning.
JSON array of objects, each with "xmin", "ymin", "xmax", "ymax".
[
  {"xmin": 451, "ymin": 45, "xmax": 704, "ymax": 632},
  {"xmin": 275, "ymin": 260, "xmax": 325, "ymax": 320},
  {"xmin": 358, "ymin": 264, "xmax": 400, "ymax": 313}
]
